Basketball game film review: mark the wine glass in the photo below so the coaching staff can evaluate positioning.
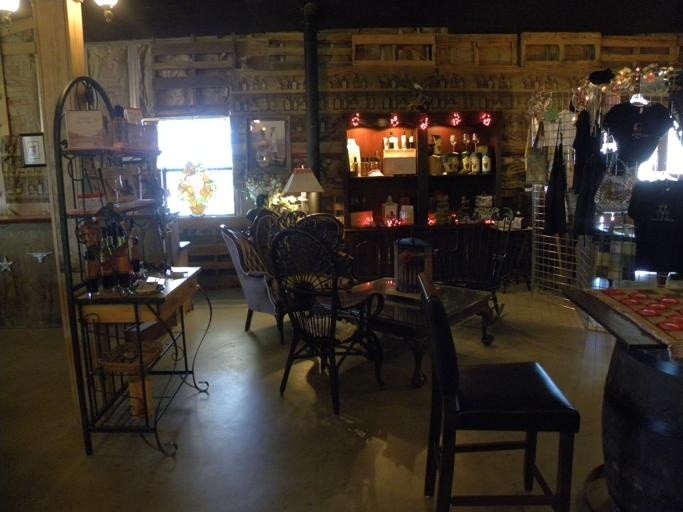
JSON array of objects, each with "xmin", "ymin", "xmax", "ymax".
[
  {"xmin": 471, "ymin": 133, "xmax": 480, "ymax": 152},
  {"xmin": 463, "ymin": 133, "xmax": 471, "ymax": 151},
  {"xmin": 111, "ymin": 175, "xmax": 122, "ymax": 206},
  {"xmin": 449, "ymin": 135, "xmax": 457, "ymax": 152}
]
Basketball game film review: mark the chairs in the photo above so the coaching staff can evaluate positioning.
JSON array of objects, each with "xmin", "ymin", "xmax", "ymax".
[{"xmin": 415, "ymin": 271, "xmax": 580, "ymax": 512}]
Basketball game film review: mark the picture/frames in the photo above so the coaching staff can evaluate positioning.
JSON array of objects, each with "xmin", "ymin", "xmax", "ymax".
[
  {"xmin": 246, "ymin": 115, "xmax": 292, "ymax": 175},
  {"xmin": 19, "ymin": 133, "xmax": 46, "ymax": 167}
]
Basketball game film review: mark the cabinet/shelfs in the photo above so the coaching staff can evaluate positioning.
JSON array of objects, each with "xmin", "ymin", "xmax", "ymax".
[
  {"xmin": 150, "ymin": 34, "xmax": 236, "ymax": 114},
  {"xmin": 344, "ymin": 109, "xmax": 500, "ymax": 222},
  {"xmin": 172, "ymin": 241, "xmax": 195, "ymax": 314},
  {"xmin": 54, "ymin": 77, "xmax": 212, "ymax": 458},
  {"xmin": 230, "ymin": 87, "xmax": 579, "ymax": 191}
]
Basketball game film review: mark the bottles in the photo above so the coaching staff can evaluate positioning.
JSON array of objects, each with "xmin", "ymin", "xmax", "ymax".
[
  {"xmin": 402, "ymin": 130, "xmax": 407, "ymax": 148},
  {"xmin": 389, "ymin": 132, "xmax": 394, "ymax": 149},
  {"xmin": 83, "ymin": 239, "xmax": 140, "ymax": 292},
  {"xmin": 444, "ymin": 152, "xmax": 492, "ymax": 174},
  {"xmin": 409, "ymin": 131, "xmax": 414, "ymax": 148},
  {"xmin": 433, "ymin": 135, "xmax": 441, "ymax": 155},
  {"xmin": 352, "ymin": 156, "xmax": 380, "ymax": 177}
]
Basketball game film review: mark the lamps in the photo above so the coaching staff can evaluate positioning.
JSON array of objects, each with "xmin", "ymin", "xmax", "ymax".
[
  {"xmin": 0, "ymin": 1, "xmax": 22, "ymax": 28},
  {"xmin": 280, "ymin": 164, "xmax": 325, "ymax": 212},
  {"xmin": 94, "ymin": 1, "xmax": 118, "ymax": 24}
]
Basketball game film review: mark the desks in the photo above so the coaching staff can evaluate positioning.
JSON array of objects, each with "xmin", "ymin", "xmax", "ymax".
[
  {"xmin": 490, "ymin": 225, "xmax": 532, "ymax": 293},
  {"xmin": 317, "ymin": 278, "xmax": 495, "ymax": 388},
  {"xmin": 562, "ymin": 286, "xmax": 682, "ymax": 510}
]
